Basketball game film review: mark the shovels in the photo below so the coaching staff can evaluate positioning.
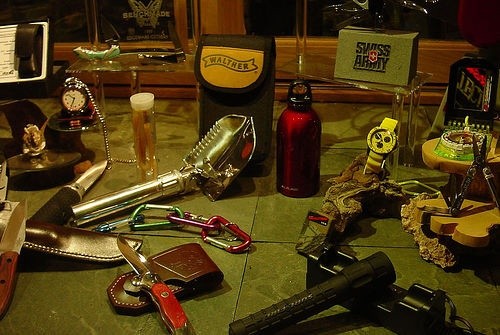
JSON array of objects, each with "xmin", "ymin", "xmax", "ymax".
[{"xmin": 68, "ymin": 113, "xmax": 257, "ymax": 229}]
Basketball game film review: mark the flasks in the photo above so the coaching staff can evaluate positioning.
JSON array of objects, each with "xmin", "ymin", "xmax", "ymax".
[{"xmin": 276, "ymin": 80, "xmax": 323, "ymax": 198}]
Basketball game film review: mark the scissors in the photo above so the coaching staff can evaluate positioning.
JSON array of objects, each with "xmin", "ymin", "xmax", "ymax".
[{"xmin": 448, "ymin": 132, "xmax": 500, "ymax": 216}]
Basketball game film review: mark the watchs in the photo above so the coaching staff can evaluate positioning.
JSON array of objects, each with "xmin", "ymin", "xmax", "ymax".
[{"xmin": 364, "ymin": 118, "xmax": 399, "ymax": 176}]
